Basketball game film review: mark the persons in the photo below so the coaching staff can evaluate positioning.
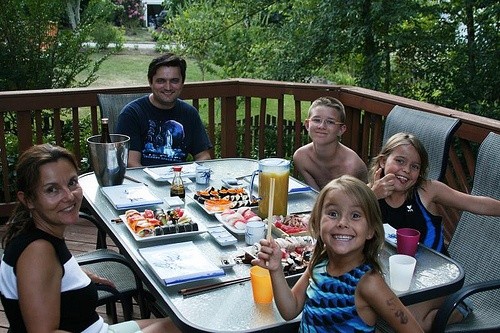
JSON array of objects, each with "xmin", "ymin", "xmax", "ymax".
[
  {"xmin": 0, "ymin": 143, "xmax": 183, "ymax": 333},
  {"xmin": 365, "ymin": 132, "xmax": 500, "ymax": 332},
  {"xmin": 116, "ymin": 54, "xmax": 213, "ymax": 168},
  {"xmin": 251, "ymin": 175, "xmax": 424, "ymax": 333},
  {"xmin": 293, "ymin": 96, "xmax": 369, "ymax": 193}
]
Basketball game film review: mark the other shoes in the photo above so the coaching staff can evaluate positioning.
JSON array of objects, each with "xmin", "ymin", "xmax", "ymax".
[{"xmin": 456, "ymin": 298, "xmax": 474, "ymax": 320}]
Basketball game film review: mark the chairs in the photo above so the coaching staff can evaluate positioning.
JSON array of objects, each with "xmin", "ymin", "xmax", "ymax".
[
  {"xmin": 97, "ymin": 93, "xmax": 149, "ymax": 248},
  {"xmin": 376, "ymin": 132, "xmax": 500, "ymax": 333},
  {"xmin": 381, "ymin": 105, "xmax": 463, "ymax": 180},
  {"xmin": 0, "ymin": 211, "xmax": 150, "ymax": 323}
]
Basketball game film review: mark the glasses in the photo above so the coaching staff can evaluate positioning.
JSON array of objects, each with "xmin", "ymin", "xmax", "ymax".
[{"xmin": 306, "ymin": 116, "xmax": 345, "ymax": 128}]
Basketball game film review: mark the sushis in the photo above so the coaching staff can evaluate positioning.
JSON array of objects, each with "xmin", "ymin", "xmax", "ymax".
[
  {"xmin": 126, "ymin": 208, "xmax": 198, "ymax": 238},
  {"xmin": 194, "ymin": 186, "xmax": 258, "ymax": 211},
  {"xmin": 221, "ymin": 207, "xmax": 262, "ymax": 230},
  {"xmin": 252, "ymin": 236, "xmax": 318, "ymax": 276}
]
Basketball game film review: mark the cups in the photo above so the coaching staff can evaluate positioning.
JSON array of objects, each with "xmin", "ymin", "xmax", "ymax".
[
  {"xmin": 196, "ymin": 165, "xmax": 211, "ymax": 185},
  {"xmin": 249, "ymin": 266, "xmax": 272, "ymax": 304},
  {"xmin": 244, "ymin": 221, "xmax": 268, "ymax": 245},
  {"xmin": 389, "ymin": 255, "xmax": 417, "ymax": 292},
  {"xmin": 396, "ymin": 228, "xmax": 421, "ymax": 257}
]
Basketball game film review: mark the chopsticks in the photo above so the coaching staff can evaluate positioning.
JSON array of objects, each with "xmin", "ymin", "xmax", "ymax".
[
  {"xmin": 266, "ymin": 177, "xmax": 276, "ymax": 242},
  {"xmin": 124, "ymin": 175, "xmax": 149, "ymax": 186},
  {"xmin": 177, "ymin": 277, "xmax": 251, "ymax": 296}
]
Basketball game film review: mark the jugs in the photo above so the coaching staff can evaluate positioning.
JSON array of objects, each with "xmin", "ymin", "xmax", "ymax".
[{"xmin": 248, "ymin": 157, "xmax": 292, "ymax": 219}]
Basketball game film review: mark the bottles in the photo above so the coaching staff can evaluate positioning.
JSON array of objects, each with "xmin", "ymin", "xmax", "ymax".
[
  {"xmin": 170, "ymin": 166, "xmax": 186, "ymax": 198},
  {"xmin": 100, "ymin": 118, "xmax": 112, "ymax": 143}
]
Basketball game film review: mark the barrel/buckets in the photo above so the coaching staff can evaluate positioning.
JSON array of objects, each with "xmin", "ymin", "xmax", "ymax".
[{"xmin": 87, "ymin": 134, "xmax": 130, "ymax": 187}]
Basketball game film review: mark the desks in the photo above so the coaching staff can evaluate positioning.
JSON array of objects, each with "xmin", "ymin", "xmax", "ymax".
[{"xmin": 78, "ymin": 158, "xmax": 465, "ymax": 333}]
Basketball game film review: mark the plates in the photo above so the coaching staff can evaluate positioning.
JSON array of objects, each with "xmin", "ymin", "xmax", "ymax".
[
  {"xmin": 382, "ymin": 222, "xmax": 398, "ymax": 248},
  {"xmin": 195, "ymin": 173, "xmax": 313, "ymax": 246},
  {"xmin": 242, "ymin": 236, "xmax": 317, "ymax": 280},
  {"xmin": 168, "ymin": 176, "xmax": 193, "ymax": 188},
  {"xmin": 212, "ymin": 254, "xmax": 236, "ymax": 270},
  {"xmin": 98, "ymin": 182, "xmax": 163, "ymax": 211},
  {"xmin": 117, "ymin": 209, "xmax": 208, "ymax": 242},
  {"xmin": 164, "ymin": 195, "xmax": 185, "ymax": 207},
  {"xmin": 142, "ymin": 161, "xmax": 201, "ymax": 182},
  {"xmin": 137, "ymin": 241, "xmax": 226, "ymax": 287}
]
